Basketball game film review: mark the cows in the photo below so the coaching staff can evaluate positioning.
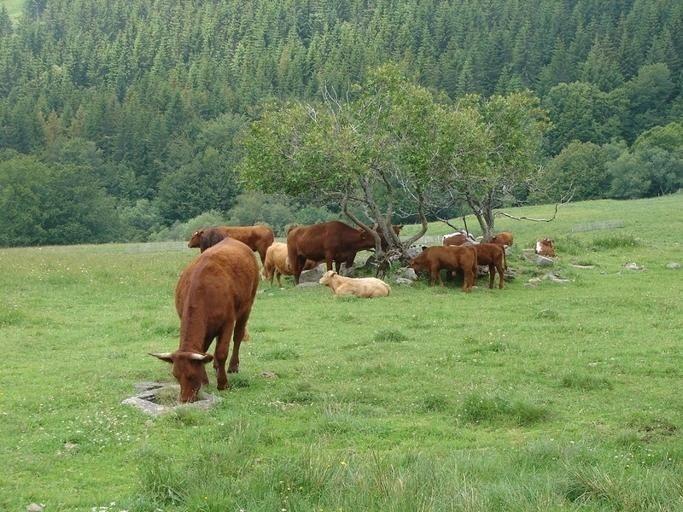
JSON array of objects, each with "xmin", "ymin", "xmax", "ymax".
[
  {"xmin": 443, "ymin": 234, "xmax": 467, "ymax": 247},
  {"xmin": 287, "ymin": 220, "xmax": 377, "ymax": 286},
  {"xmin": 319, "ymin": 269, "xmax": 392, "ymax": 298},
  {"xmin": 535, "ymin": 237, "xmax": 555, "ymax": 257},
  {"xmin": 410, "ymin": 246, "xmax": 478, "ymax": 293},
  {"xmin": 490, "ymin": 231, "xmax": 514, "ymax": 246},
  {"xmin": 356, "ymin": 222, "xmax": 404, "ymax": 246},
  {"xmin": 187, "ymin": 223, "xmax": 274, "ymax": 267},
  {"xmin": 262, "ymin": 242, "xmax": 322, "ymax": 290},
  {"xmin": 148, "ymin": 237, "xmax": 261, "ymax": 403},
  {"xmin": 447, "ymin": 242, "xmax": 508, "ymax": 289}
]
